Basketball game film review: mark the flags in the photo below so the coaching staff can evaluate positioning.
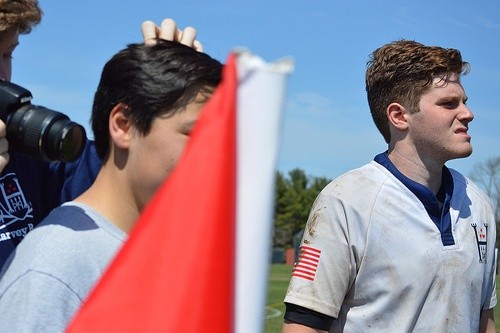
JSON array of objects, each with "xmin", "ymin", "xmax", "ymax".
[{"xmin": 63, "ymin": 41, "xmax": 294, "ymax": 333}]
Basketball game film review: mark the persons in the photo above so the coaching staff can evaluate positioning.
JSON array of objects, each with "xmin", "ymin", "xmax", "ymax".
[
  {"xmin": 0, "ymin": 0, "xmax": 202, "ymax": 271},
  {"xmin": 0, "ymin": 39, "xmax": 223, "ymax": 333},
  {"xmin": 282, "ymin": 40, "xmax": 500, "ymax": 333}
]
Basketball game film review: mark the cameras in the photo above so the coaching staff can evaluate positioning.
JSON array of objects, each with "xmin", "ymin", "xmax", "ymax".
[{"xmin": 0, "ymin": 79, "xmax": 86, "ymax": 163}]
